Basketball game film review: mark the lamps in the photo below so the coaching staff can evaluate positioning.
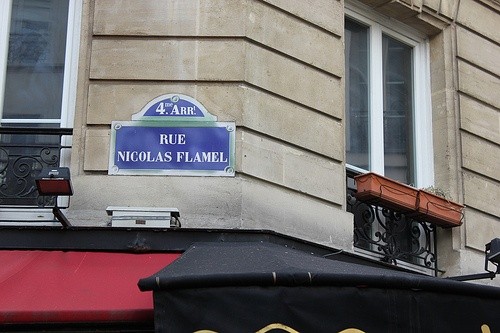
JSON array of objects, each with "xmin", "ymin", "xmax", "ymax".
[
  {"xmin": 441, "ymin": 237, "xmax": 500, "ymax": 284},
  {"xmin": 34, "ymin": 166, "xmax": 75, "ymax": 228}
]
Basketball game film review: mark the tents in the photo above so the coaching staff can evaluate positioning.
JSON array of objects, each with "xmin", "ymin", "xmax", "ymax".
[{"xmin": 138, "ymin": 242, "xmax": 500, "ymax": 333}]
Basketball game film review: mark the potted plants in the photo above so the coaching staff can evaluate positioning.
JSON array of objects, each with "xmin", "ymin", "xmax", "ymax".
[{"xmin": 352, "ymin": 170, "xmax": 466, "ymax": 227}]
